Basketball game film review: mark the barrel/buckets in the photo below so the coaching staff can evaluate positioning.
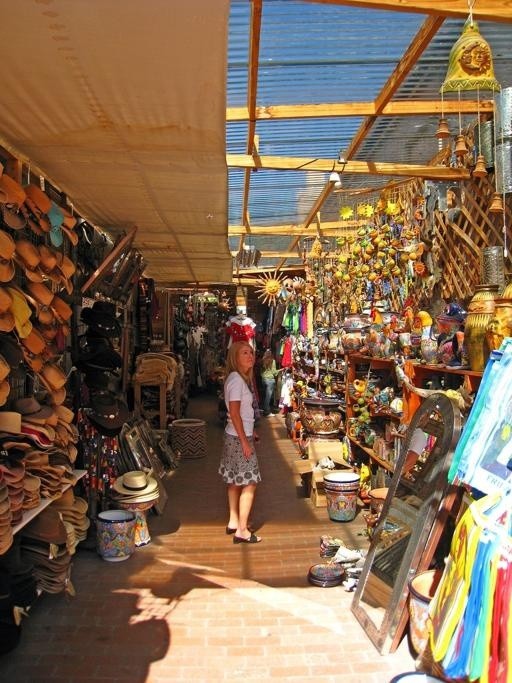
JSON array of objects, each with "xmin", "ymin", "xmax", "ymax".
[
  {"xmin": 407, "ymin": 569, "xmax": 446, "ymax": 655},
  {"xmin": 94, "ymin": 509, "xmax": 135, "ymax": 562}
]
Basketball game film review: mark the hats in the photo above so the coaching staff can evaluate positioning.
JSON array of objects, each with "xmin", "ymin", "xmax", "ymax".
[
  {"xmin": 109, "ymin": 470, "xmax": 160, "ymax": 512},
  {"xmin": 76, "ymin": 301, "xmax": 128, "ymax": 431},
  {"xmin": 0, "ymin": 175, "xmax": 90, "ymax": 654}
]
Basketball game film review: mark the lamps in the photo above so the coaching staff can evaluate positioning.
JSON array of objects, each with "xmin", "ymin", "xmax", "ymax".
[{"xmin": 329, "ymin": 149, "xmax": 349, "ymax": 189}]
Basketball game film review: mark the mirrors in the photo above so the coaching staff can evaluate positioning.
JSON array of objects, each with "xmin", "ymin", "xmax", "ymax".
[{"xmin": 348, "ymin": 391, "xmax": 463, "ymax": 655}]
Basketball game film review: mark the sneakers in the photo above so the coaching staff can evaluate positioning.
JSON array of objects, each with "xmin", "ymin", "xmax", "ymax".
[{"xmin": 259, "ymin": 409, "xmax": 275, "ymax": 417}]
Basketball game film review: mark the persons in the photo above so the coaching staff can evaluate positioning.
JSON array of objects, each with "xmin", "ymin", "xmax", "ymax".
[{"xmin": 218, "ymin": 340, "xmax": 261, "ymax": 544}]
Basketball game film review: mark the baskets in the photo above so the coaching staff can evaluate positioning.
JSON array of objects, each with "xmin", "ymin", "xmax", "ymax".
[{"xmin": 300, "ymin": 409, "xmax": 342, "ymax": 432}]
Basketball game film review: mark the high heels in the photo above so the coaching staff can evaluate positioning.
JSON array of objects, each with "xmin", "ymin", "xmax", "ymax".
[{"xmin": 226, "ymin": 525, "xmax": 262, "ymax": 544}]
